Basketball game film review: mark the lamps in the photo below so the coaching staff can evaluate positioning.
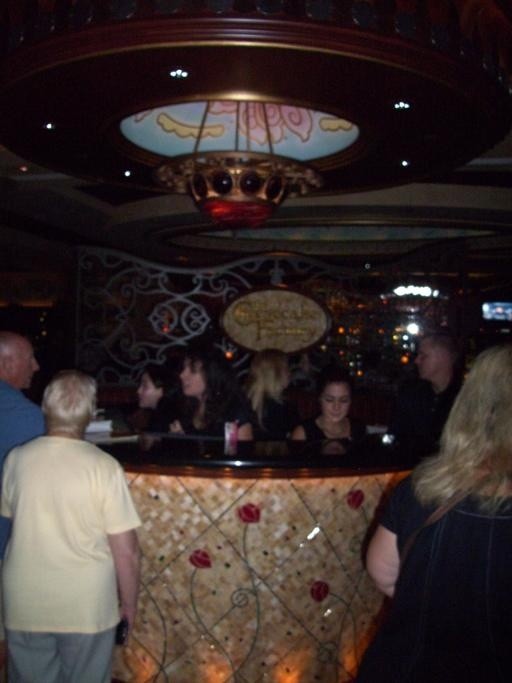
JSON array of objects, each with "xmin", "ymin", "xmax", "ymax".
[{"xmin": 117, "ymin": 90, "xmax": 363, "ymax": 232}]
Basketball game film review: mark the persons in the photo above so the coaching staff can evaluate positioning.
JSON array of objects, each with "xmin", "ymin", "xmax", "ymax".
[
  {"xmin": 1, "ymin": 328, "xmax": 50, "ymax": 552},
  {"xmin": 394, "ymin": 333, "xmax": 468, "ymax": 451},
  {"xmin": 231, "ymin": 349, "xmax": 300, "ymax": 430},
  {"xmin": 355, "ymin": 347, "xmax": 511, "ymax": 682},
  {"xmin": 140, "ymin": 365, "xmax": 168, "ymax": 431},
  {"xmin": 300, "ymin": 348, "xmax": 329, "ymax": 391},
  {"xmin": 166, "ymin": 348, "xmax": 253, "ymax": 441},
  {"xmin": 290, "ymin": 369, "xmax": 367, "ymax": 439},
  {"xmin": 2, "ymin": 371, "xmax": 138, "ymax": 682}
]
